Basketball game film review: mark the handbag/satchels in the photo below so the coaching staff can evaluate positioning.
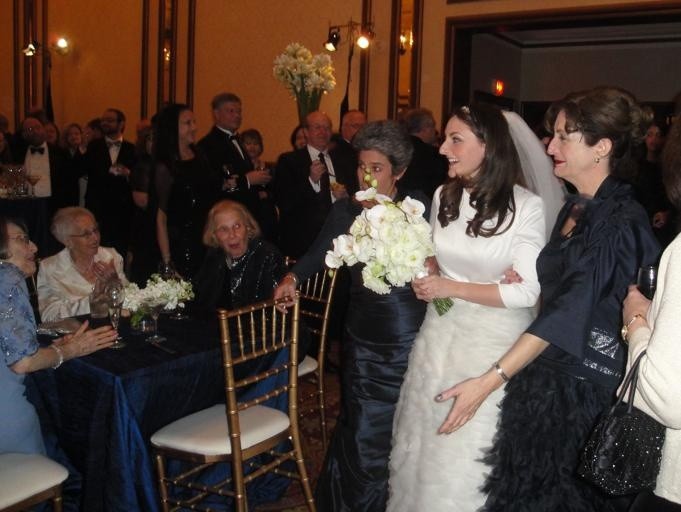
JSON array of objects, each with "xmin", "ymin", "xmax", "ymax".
[{"xmin": 576, "ymin": 350, "xmax": 667, "ymax": 499}]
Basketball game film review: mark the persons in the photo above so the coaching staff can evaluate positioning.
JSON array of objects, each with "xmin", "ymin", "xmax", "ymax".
[
  {"xmin": 290, "ymin": 124, "xmax": 309, "ymax": 151},
  {"xmin": 0, "ymin": 114, "xmax": 20, "ymax": 219},
  {"xmin": 148, "ymin": 103, "xmax": 219, "ymax": 280},
  {"xmin": 43, "ymin": 121, "xmax": 61, "ymax": 146},
  {"xmin": 635, "ymin": 116, "xmax": 678, "ymax": 248},
  {"xmin": 395, "ymin": 106, "xmax": 449, "ymax": 199},
  {"xmin": 275, "ymin": 109, "xmax": 358, "ymax": 266},
  {"xmin": 272, "ymin": 119, "xmax": 432, "ymax": 512},
  {"xmin": 338, "ymin": 110, "xmax": 367, "ymax": 144},
  {"xmin": 82, "ymin": 116, "xmax": 103, "ymax": 140},
  {"xmin": 184, "ymin": 199, "xmax": 283, "ymax": 324},
  {"xmin": 12, "ymin": 116, "xmax": 81, "ymax": 258},
  {"xmin": 72, "ymin": 108, "xmax": 137, "ymax": 258},
  {"xmin": 0, "ymin": 215, "xmax": 120, "ymax": 510},
  {"xmin": 614, "ymin": 115, "xmax": 681, "ymax": 512},
  {"xmin": 239, "ymin": 128, "xmax": 283, "ymax": 227},
  {"xmin": 127, "ymin": 120, "xmax": 157, "ymax": 289},
  {"xmin": 433, "ymin": 87, "xmax": 663, "ymax": 511},
  {"xmin": 198, "ymin": 91, "xmax": 274, "ymax": 226},
  {"xmin": 62, "ymin": 123, "xmax": 83, "ymax": 162},
  {"xmin": 35, "ymin": 206, "xmax": 129, "ymax": 324},
  {"xmin": 383, "ymin": 100, "xmax": 568, "ymax": 512}
]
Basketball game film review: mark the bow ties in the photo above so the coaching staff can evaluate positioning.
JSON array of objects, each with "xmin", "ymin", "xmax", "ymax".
[
  {"xmin": 230, "ymin": 135, "xmax": 239, "ymax": 140},
  {"xmin": 107, "ymin": 141, "xmax": 121, "ymax": 147},
  {"xmin": 31, "ymin": 147, "xmax": 45, "ymax": 154}
]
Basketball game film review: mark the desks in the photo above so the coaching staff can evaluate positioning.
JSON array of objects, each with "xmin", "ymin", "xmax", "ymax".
[
  {"xmin": 0, "ymin": 188, "xmax": 66, "ymax": 268},
  {"xmin": 22, "ymin": 308, "xmax": 287, "ymax": 510}
]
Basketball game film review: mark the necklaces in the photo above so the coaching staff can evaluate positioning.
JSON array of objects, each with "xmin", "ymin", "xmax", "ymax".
[
  {"xmin": 230, "ymin": 244, "xmax": 258, "ymax": 294},
  {"xmin": 73, "ymin": 258, "xmax": 94, "ymax": 275},
  {"xmin": 230, "ymin": 255, "xmax": 246, "ymax": 267}
]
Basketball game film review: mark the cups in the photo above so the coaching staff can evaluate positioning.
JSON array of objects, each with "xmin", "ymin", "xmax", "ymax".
[
  {"xmin": 89, "ymin": 286, "xmax": 109, "ymax": 320},
  {"xmin": 159, "ymin": 263, "xmax": 175, "ymax": 281},
  {"xmin": 637, "ymin": 266, "xmax": 658, "ymax": 302}
]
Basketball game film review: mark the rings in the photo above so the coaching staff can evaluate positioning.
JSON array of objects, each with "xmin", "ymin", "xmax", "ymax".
[{"xmin": 100, "ymin": 272, "xmax": 104, "ymax": 276}]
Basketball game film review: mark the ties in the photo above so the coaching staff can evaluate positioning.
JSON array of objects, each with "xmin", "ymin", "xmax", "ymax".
[{"xmin": 318, "ymin": 153, "xmax": 332, "ymax": 204}]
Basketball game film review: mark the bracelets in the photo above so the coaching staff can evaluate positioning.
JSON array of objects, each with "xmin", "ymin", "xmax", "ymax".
[
  {"xmin": 47, "ymin": 344, "xmax": 64, "ymax": 371},
  {"xmin": 620, "ymin": 314, "xmax": 647, "ymax": 344},
  {"xmin": 282, "ymin": 275, "xmax": 298, "ymax": 286},
  {"xmin": 495, "ymin": 362, "xmax": 510, "ymax": 382}
]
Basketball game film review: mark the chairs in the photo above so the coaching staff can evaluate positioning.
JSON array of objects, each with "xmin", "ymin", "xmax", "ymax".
[
  {"xmin": 280, "ymin": 255, "xmax": 338, "ymax": 457},
  {"xmin": 151, "ymin": 290, "xmax": 318, "ymax": 512},
  {"xmin": 0, "ymin": 450, "xmax": 69, "ymax": 512}
]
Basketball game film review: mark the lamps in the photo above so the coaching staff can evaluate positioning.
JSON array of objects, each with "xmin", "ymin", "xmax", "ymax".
[
  {"xmin": 322, "ymin": 16, "xmax": 375, "ymax": 133},
  {"xmin": 21, "ymin": 36, "xmax": 70, "ymax": 126}
]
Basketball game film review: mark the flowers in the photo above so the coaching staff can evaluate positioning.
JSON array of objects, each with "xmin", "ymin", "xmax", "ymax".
[
  {"xmin": 270, "ymin": 42, "xmax": 338, "ymax": 127},
  {"xmin": 116, "ymin": 273, "xmax": 193, "ymax": 325},
  {"xmin": 322, "ymin": 164, "xmax": 453, "ymax": 316}
]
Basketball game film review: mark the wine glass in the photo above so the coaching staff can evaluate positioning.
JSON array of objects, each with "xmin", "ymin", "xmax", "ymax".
[
  {"xmin": 25, "ymin": 175, "xmax": 41, "ymax": 200},
  {"xmin": 253, "ymin": 156, "xmax": 268, "ymax": 188},
  {"xmin": 102, "ymin": 283, "xmax": 127, "ymax": 350},
  {"xmin": 142, "ymin": 299, "xmax": 169, "ymax": 344},
  {"xmin": 223, "ymin": 165, "xmax": 241, "ymax": 192}
]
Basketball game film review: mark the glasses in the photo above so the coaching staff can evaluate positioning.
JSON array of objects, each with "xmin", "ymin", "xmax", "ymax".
[
  {"xmin": 6, "ymin": 235, "xmax": 30, "ymax": 245},
  {"xmin": 67, "ymin": 228, "xmax": 99, "ymax": 237},
  {"xmin": 100, "ymin": 117, "xmax": 117, "ymax": 123},
  {"xmin": 24, "ymin": 124, "xmax": 41, "ymax": 135}
]
